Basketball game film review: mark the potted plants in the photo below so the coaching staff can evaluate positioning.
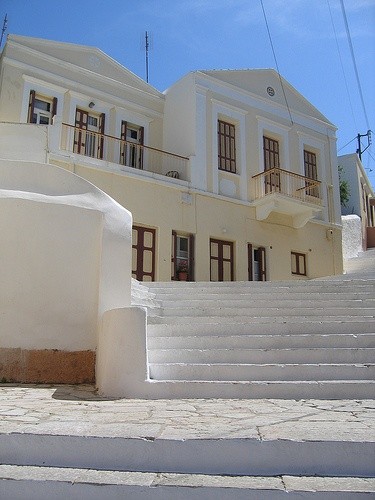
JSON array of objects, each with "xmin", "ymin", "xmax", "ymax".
[{"xmin": 177, "ymin": 261, "xmax": 189, "ymax": 280}]
